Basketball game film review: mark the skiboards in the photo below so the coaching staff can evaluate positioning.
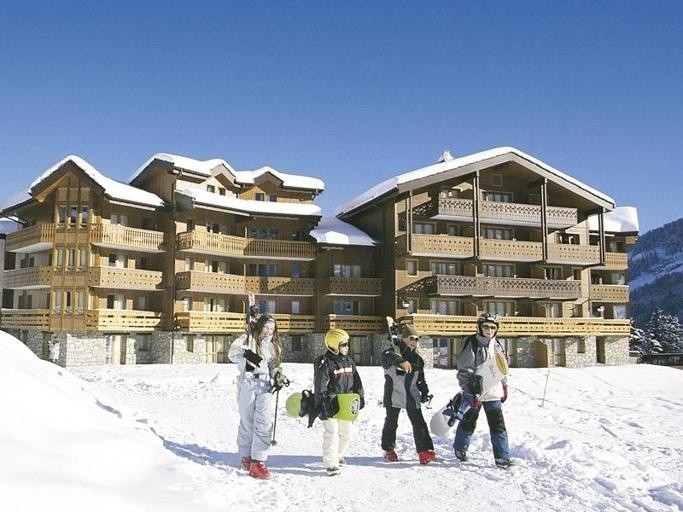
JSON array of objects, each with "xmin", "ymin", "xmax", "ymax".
[
  {"xmin": 244, "ymin": 293, "xmax": 258, "ymax": 379},
  {"xmin": 386, "ymin": 316, "xmax": 405, "ymax": 372}
]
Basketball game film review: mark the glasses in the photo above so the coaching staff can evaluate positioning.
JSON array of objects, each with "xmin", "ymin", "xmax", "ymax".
[
  {"xmin": 409, "ymin": 336, "xmax": 419, "ymax": 342},
  {"xmin": 482, "ymin": 324, "xmax": 496, "ymax": 330},
  {"xmin": 340, "ymin": 342, "xmax": 348, "ymax": 347}
]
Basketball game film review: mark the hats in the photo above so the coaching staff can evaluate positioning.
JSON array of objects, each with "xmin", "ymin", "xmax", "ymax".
[{"xmin": 402, "ymin": 324, "xmax": 418, "ymax": 338}]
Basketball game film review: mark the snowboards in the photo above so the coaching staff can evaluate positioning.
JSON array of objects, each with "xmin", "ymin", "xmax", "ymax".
[
  {"xmin": 286, "ymin": 393, "xmax": 360, "ymax": 421},
  {"xmin": 431, "ymin": 351, "xmax": 508, "ymax": 435}
]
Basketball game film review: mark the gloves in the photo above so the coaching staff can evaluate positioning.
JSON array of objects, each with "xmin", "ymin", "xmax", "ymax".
[
  {"xmin": 468, "ymin": 400, "xmax": 481, "ymax": 410},
  {"xmin": 501, "ymin": 384, "xmax": 508, "ymax": 403}
]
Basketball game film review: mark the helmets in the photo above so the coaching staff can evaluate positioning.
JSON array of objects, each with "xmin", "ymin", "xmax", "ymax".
[
  {"xmin": 324, "ymin": 328, "xmax": 351, "ymax": 356},
  {"xmin": 477, "ymin": 312, "xmax": 499, "ymax": 337}
]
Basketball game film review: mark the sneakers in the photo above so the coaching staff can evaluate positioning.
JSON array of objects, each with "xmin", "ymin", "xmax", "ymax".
[
  {"xmin": 249, "ymin": 460, "xmax": 271, "ymax": 479},
  {"xmin": 326, "ymin": 466, "xmax": 340, "ymax": 476},
  {"xmin": 418, "ymin": 451, "xmax": 436, "ymax": 465},
  {"xmin": 239, "ymin": 458, "xmax": 251, "ymax": 471},
  {"xmin": 454, "ymin": 448, "xmax": 467, "ymax": 461},
  {"xmin": 385, "ymin": 450, "xmax": 399, "ymax": 461},
  {"xmin": 340, "ymin": 459, "xmax": 347, "ymax": 466},
  {"xmin": 495, "ymin": 458, "xmax": 511, "ymax": 469}
]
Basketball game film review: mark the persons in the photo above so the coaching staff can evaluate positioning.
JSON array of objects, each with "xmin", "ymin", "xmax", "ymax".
[
  {"xmin": 227, "ymin": 313, "xmax": 281, "ymax": 480},
  {"xmin": 380, "ymin": 324, "xmax": 437, "ymax": 465},
  {"xmin": 315, "ymin": 327, "xmax": 365, "ymax": 477},
  {"xmin": 451, "ymin": 313, "xmax": 515, "ymax": 471}
]
